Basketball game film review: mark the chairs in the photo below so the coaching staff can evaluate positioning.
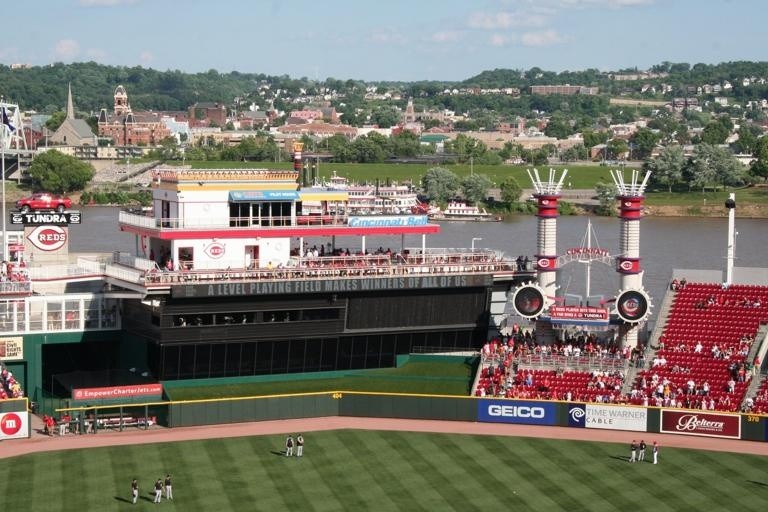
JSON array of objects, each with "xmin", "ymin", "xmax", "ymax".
[{"xmin": 478, "ymin": 281, "xmax": 767, "ymax": 418}]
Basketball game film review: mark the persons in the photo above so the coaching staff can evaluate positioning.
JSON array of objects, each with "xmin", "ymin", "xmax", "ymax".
[
  {"xmin": 285, "ymin": 435, "xmax": 294, "ymax": 457},
  {"xmin": 146, "ymin": 244, "xmax": 192, "ymax": 271},
  {"xmin": 652, "ymin": 441, "xmax": 658, "ymax": 465},
  {"xmin": 165, "ymin": 475, "xmax": 174, "ymax": 501},
  {"xmin": 267, "ymin": 239, "xmax": 504, "ymax": 272},
  {"xmin": 0, "ymin": 363, "xmax": 24, "ymax": 400},
  {"xmin": 296, "ymin": 433, "xmax": 304, "ymax": 456},
  {"xmin": 0, "ymin": 252, "xmax": 29, "ymax": 291},
  {"xmin": 479, "ymin": 277, "xmax": 767, "ymax": 414},
  {"xmin": 132, "ymin": 478, "xmax": 138, "ymax": 504},
  {"xmin": 175, "ymin": 316, "xmax": 205, "ymax": 328},
  {"xmin": 47, "ymin": 304, "xmax": 118, "ymax": 330},
  {"xmin": 42, "ymin": 412, "xmax": 95, "ymax": 437},
  {"xmin": 267, "ymin": 311, "xmax": 292, "ymax": 322},
  {"xmin": 638, "ymin": 440, "xmax": 647, "ymax": 461},
  {"xmin": 516, "ymin": 254, "xmax": 530, "ymax": 272},
  {"xmin": 153, "ymin": 478, "xmax": 165, "ymax": 503},
  {"xmin": 223, "ymin": 314, "xmax": 248, "ymax": 324},
  {"xmin": 628, "ymin": 440, "xmax": 638, "ymax": 463}
]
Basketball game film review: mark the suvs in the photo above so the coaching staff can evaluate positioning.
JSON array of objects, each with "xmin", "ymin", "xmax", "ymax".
[{"xmin": 14, "ymin": 191, "xmax": 72, "ymax": 214}]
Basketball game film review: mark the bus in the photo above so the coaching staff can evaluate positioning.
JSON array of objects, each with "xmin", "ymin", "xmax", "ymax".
[{"xmin": 729, "ymin": 153, "xmax": 759, "ymax": 167}]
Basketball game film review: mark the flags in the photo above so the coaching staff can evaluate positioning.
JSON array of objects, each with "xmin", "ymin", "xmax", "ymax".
[{"xmin": 2, "ymin": 106, "xmax": 17, "ymax": 136}]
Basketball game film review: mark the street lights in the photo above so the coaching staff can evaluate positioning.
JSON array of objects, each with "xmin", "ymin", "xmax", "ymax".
[{"xmin": 724, "ymin": 192, "xmax": 738, "ymax": 288}]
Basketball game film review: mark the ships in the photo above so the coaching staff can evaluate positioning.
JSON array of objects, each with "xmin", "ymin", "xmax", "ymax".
[{"xmin": 301, "ymin": 162, "xmax": 440, "ymax": 217}]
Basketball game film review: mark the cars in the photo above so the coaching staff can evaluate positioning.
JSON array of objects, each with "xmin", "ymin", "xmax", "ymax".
[
  {"xmin": 592, "ymin": 156, "xmax": 627, "ymax": 167},
  {"xmin": 683, "ymin": 154, "xmax": 699, "ymax": 165},
  {"xmin": 505, "ymin": 156, "xmax": 530, "ymax": 164}
]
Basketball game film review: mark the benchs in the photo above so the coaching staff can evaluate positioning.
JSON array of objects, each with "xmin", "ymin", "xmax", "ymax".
[{"xmin": 103, "ymin": 421, "xmax": 149, "ymax": 430}]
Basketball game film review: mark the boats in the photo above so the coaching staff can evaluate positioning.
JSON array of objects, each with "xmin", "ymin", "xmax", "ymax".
[{"xmin": 428, "ymin": 197, "xmax": 504, "ymax": 224}]
